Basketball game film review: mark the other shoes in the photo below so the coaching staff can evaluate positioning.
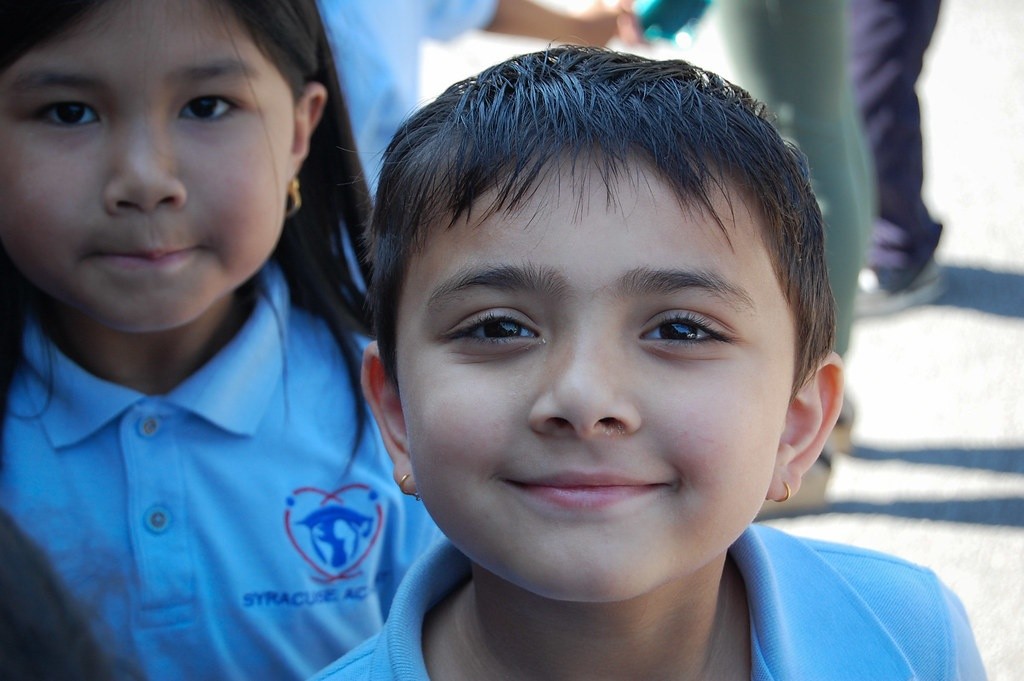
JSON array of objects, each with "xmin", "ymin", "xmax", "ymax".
[{"xmin": 853, "ymin": 257, "xmax": 940, "ymax": 315}]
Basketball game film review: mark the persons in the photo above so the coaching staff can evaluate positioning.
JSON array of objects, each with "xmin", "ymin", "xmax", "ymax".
[
  {"xmin": 612, "ymin": 0, "xmax": 874, "ymax": 519},
  {"xmin": 0, "ymin": 0, "xmax": 443, "ymax": 679},
  {"xmin": 314, "ymin": 0, "xmax": 615, "ymax": 309},
  {"xmin": 307, "ymin": 44, "xmax": 987, "ymax": 678},
  {"xmin": 845, "ymin": 3, "xmax": 952, "ymax": 325}
]
